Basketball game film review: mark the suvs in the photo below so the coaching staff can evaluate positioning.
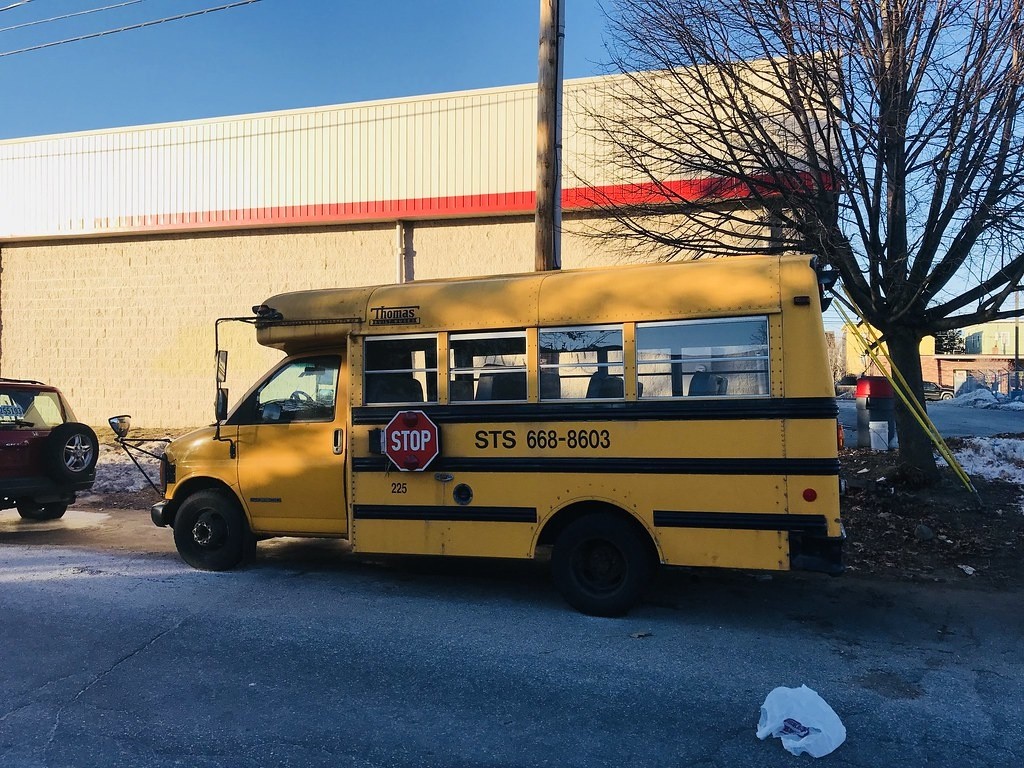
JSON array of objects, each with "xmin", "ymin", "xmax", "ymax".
[{"xmin": 0, "ymin": 377, "xmax": 99, "ymax": 522}]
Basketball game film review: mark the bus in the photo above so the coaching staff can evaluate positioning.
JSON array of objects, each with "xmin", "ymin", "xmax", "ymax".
[{"xmin": 109, "ymin": 253, "xmax": 848, "ymax": 617}]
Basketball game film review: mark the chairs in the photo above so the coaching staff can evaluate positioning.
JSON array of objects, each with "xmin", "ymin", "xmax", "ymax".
[{"xmin": 367, "ymin": 365, "xmax": 731, "ymax": 405}]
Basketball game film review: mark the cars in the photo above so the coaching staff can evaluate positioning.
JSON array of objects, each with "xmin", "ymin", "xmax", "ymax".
[{"xmin": 921, "ymin": 381, "xmax": 954, "ymax": 401}]
